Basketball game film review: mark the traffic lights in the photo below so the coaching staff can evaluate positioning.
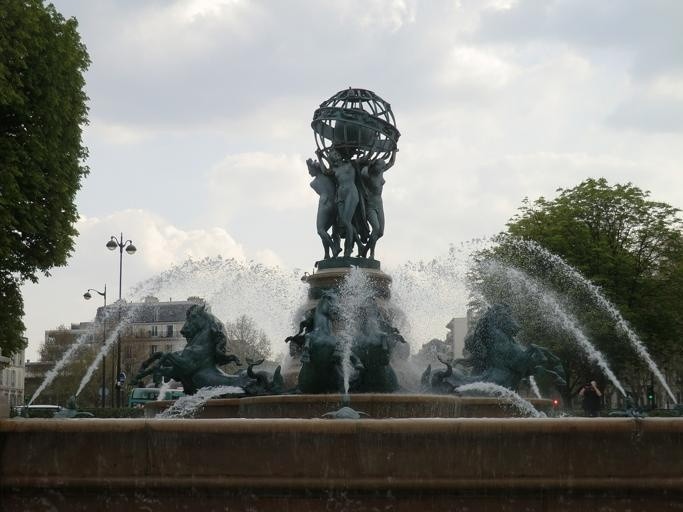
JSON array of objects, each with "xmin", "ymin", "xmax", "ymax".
[
  {"xmin": 646, "ymin": 386, "xmax": 652, "ymax": 401},
  {"xmin": 552, "ymin": 399, "xmax": 559, "ymax": 410}
]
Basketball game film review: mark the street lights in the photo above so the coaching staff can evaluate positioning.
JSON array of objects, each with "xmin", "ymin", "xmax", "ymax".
[
  {"xmin": 106, "ymin": 234, "xmax": 136, "ymax": 407},
  {"xmin": 82, "ymin": 285, "xmax": 106, "ymax": 408}
]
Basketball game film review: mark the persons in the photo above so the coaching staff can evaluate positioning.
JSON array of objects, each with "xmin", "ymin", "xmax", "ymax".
[
  {"xmin": 578, "ymin": 377, "xmax": 604, "ymax": 417},
  {"xmin": 305, "ymin": 141, "xmax": 397, "ymax": 260}
]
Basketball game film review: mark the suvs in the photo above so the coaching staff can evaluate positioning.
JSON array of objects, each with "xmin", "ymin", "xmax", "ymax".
[{"xmin": 10, "ymin": 404, "xmax": 93, "ymax": 417}]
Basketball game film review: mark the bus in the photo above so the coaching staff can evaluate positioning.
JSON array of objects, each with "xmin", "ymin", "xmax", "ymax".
[{"xmin": 129, "ymin": 389, "xmax": 185, "ymax": 415}]
